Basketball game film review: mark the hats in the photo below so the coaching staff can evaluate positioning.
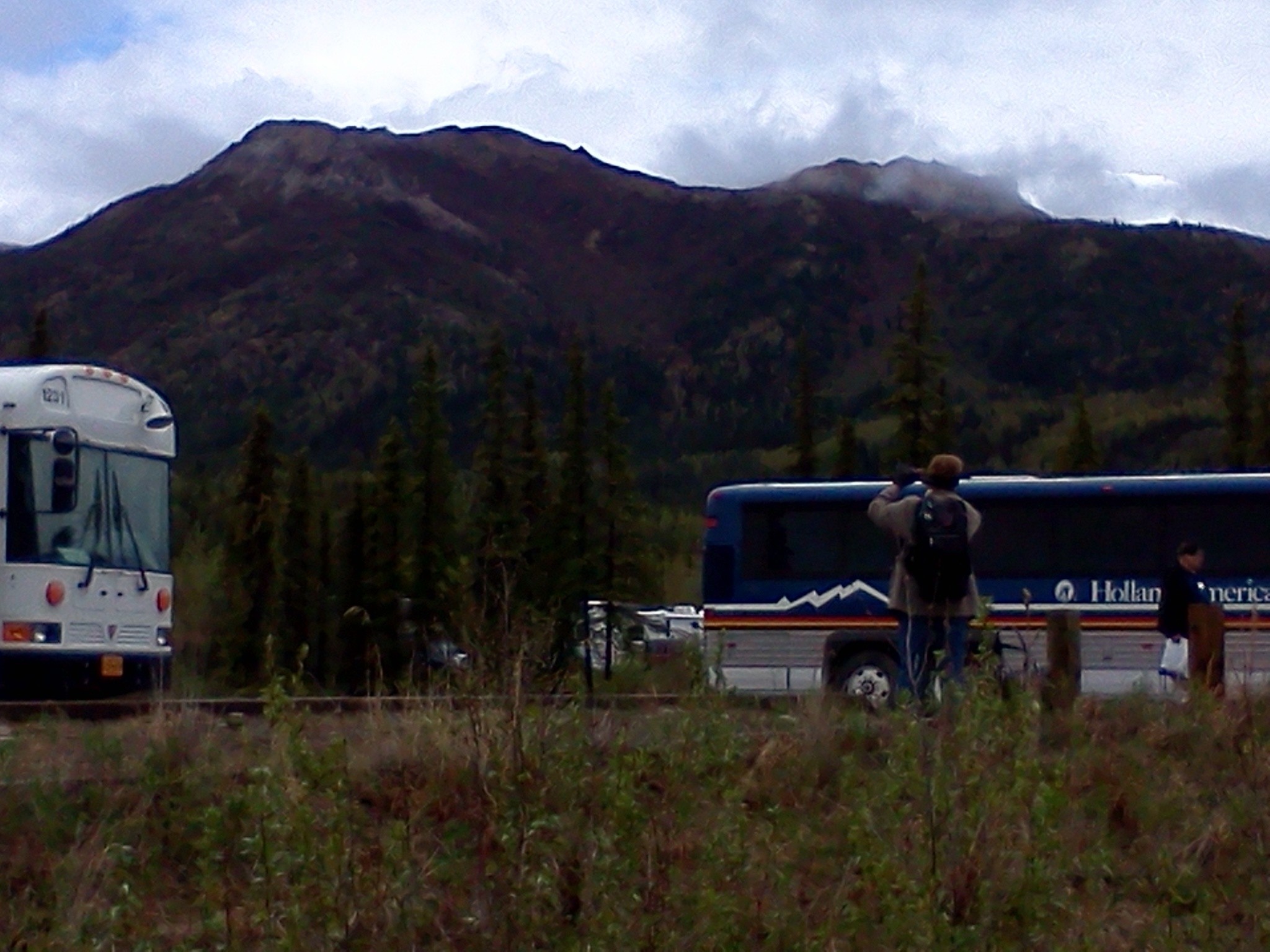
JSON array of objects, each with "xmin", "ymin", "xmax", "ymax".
[{"xmin": 929, "ymin": 455, "xmax": 963, "ymax": 475}]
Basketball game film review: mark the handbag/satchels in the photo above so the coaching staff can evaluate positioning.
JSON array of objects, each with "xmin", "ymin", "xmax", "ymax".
[{"xmin": 1159, "ymin": 636, "xmax": 1189, "ymax": 681}]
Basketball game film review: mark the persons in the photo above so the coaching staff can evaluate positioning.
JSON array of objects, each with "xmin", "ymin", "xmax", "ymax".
[
  {"xmin": 1157, "ymin": 537, "xmax": 1211, "ymax": 704},
  {"xmin": 870, "ymin": 454, "xmax": 984, "ymax": 707}
]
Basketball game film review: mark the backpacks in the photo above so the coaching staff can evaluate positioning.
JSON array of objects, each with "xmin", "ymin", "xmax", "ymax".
[{"xmin": 899, "ymin": 497, "xmax": 972, "ymax": 604}]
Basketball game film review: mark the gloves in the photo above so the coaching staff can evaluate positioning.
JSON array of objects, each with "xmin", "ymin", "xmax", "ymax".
[{"xmin": 893, "ymin": 466, "xmax": 920, "ymax": 488}]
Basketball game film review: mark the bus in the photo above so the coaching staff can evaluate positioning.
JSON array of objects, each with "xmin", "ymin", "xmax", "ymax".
[
  {"xmin": 0, "ymin": 363, "xmax": 180, "ymax": 704},
  {"xmin": 698, "ymin": 470, "xmax": 1270, "ymax": 720}
]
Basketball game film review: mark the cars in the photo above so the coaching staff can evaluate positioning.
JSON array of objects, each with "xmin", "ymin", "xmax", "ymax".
[{"xmin": 583, "ymin": 600, "xmax": 697, "ymax": 670}]
